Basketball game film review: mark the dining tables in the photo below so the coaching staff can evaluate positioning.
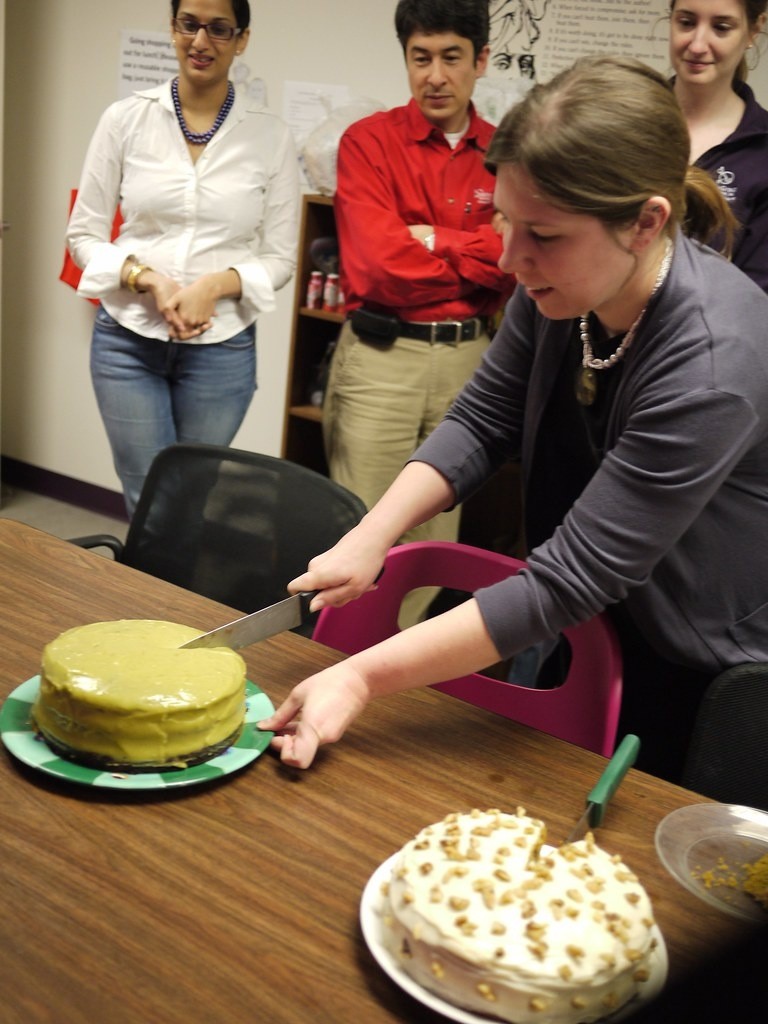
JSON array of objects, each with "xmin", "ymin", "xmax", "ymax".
[{"xmin": 0, "ymin": 518, "xmax": 768, "ymax": 1024}]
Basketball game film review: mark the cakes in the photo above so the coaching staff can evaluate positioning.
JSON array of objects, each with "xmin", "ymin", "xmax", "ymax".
[
  {"xmin": 28, "ymin": 618, "xmax": 248, "ymax": 773},
  {"xmin": 382, "ymin": 805, "xmax": 657, "ymax": 1024}
]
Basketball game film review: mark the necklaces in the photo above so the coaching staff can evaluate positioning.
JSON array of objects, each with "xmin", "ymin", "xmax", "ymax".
[
  {"xmin": 577, "ymin": 241, "xmax": 676, "ymax": 405},
  {"xmin": 173, "ymin": 76, "xmax": 234, "ymax": 144}
]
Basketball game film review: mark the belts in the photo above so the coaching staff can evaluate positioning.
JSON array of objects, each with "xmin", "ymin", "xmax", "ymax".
[{"xmin": 400, "ymin": 315, "xmax": 487, "ymax": 347}]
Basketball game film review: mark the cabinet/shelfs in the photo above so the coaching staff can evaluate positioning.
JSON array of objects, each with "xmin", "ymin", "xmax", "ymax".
[{"xmin": 279, "ymin": 189, "xmax": 348, "ymax": 481}]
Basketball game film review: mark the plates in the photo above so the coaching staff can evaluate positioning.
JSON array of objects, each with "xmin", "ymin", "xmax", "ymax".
[
  {"xmin": 360, "ymin": 851, "xmax": 669, "ymax": 1024},
  {"xmin": 0, "ymin": 674, "xmax": 276, "ymax": 790},
  {"xmin": 654, "ymin": 802, "xmax": 768, "ymax": 926}
]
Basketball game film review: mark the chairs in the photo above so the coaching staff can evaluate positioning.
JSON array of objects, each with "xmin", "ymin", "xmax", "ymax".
[
  {"xmin": 310, "ymin": 541, "xmax": 624, "ymax": 760},
  {"xmin": 63, "ymin": 441, "xmax": 370, "ymax": 641}
]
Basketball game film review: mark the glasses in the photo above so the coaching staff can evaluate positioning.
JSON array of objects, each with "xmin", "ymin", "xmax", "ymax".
[{"xmin": 172, "ymin": 18, "xmax": 242, "ymax": 40}]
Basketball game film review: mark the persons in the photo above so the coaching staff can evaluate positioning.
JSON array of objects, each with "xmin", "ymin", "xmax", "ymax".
[
  {"xmin": 321, "ymin": 0, "xmax": 516, "ymax": 547},
  {"xmin": 257, "ymin": 55, "xmax": 767, "ymax": 809},
  {"xmin": 666, "ymin": 0, "xmax": 768, "ymax": 293},
  {"xmin": 68, "ymin": 0, "xmax": 310, "ymax": 519}
]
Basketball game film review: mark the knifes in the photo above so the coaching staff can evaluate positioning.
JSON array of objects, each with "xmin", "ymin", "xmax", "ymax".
[
  {"xmin": 566, "ymin": 734, "xmax": 641, "ymax": 844},
  {"xmin": 179, "ymin": 567, "xmax": 385, "ymax": 651}
]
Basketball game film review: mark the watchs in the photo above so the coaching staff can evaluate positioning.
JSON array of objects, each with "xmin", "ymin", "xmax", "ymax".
[{"xmin": 425, "ymin": 234, "xmax": 435, "ymax": 250}]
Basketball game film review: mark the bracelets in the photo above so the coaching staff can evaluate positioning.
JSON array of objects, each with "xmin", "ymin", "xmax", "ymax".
[{"xmin": 127, "ymin": 266, "xmax": 148, "ymax": 295}]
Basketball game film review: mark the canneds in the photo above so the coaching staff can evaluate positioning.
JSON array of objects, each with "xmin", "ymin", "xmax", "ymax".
[{"xmin": 305, "ymin": 271, "xmax": 345, "ymax": 315}]
